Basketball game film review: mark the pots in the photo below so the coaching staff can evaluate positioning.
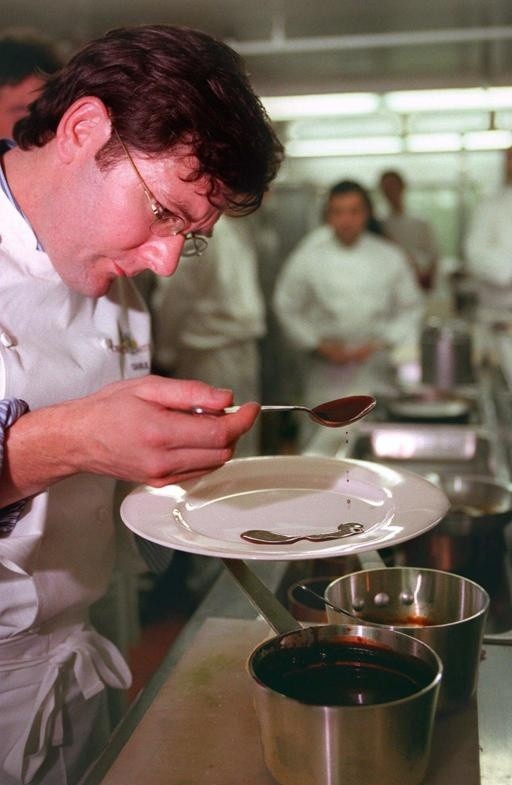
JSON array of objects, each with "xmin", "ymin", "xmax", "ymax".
[
  {"xmin": 246, "ymin": 624, "xmax": 443, "ymax": 785},
  {"xmin": 321, "ymin": 573, "xmax": 481, "ymax": 717}
]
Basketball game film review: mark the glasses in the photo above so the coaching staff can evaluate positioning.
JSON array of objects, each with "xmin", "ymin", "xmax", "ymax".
[{"xmin": 113, "ymin": 125, "xmax": 212, "ymax": 265}]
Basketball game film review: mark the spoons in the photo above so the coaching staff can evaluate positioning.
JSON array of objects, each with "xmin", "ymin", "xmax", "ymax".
[{"xmin": 194, "ymin": 396, "xmax": 379, "ymax": 427}]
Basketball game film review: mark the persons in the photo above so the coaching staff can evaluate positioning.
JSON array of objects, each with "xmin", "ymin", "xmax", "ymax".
[
  {"xmin": 272, "ymin": 182, "xmax": 427, "ymax": 442},
  {"xmin": 377, "ymin": 170, "xmax": 439, "ymax": 284},
  {"xmin": 145, "ymin": 220, "xmax": 268, "ymax": 463},
  {"xmin": 461, "ymin": 146, "xmax": 512, "ymax": 378},
  {"xmin": 3, "ymin": 38, "xmax": 54, "ymax": 141},
  {"xmin": 3, "ymin": 23, "xmax": 283, "ymax": 784}
]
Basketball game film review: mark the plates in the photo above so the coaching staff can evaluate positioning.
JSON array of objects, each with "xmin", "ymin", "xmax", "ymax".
[
  {"xmin": 388, "ymin": 395, "xmax": 476, "ymax": 422},
  {"xmin": 119, "ymin": 452, "xmax": 452, "ymax": 563}
]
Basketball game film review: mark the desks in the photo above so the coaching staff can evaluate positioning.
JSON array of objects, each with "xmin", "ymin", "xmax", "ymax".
[{"xmin": 68, "ymin": 374, "xmax": 511, "ymax": 785}]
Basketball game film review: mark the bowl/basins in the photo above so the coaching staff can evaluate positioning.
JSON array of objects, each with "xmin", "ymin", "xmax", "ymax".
[
  {"xmin": 286, "ymin": 576, "xmax": 334, "ymax": 623},
  {"xmin": 434, "ymin": 475, "xmax": 512, "ymax": 536}
]
